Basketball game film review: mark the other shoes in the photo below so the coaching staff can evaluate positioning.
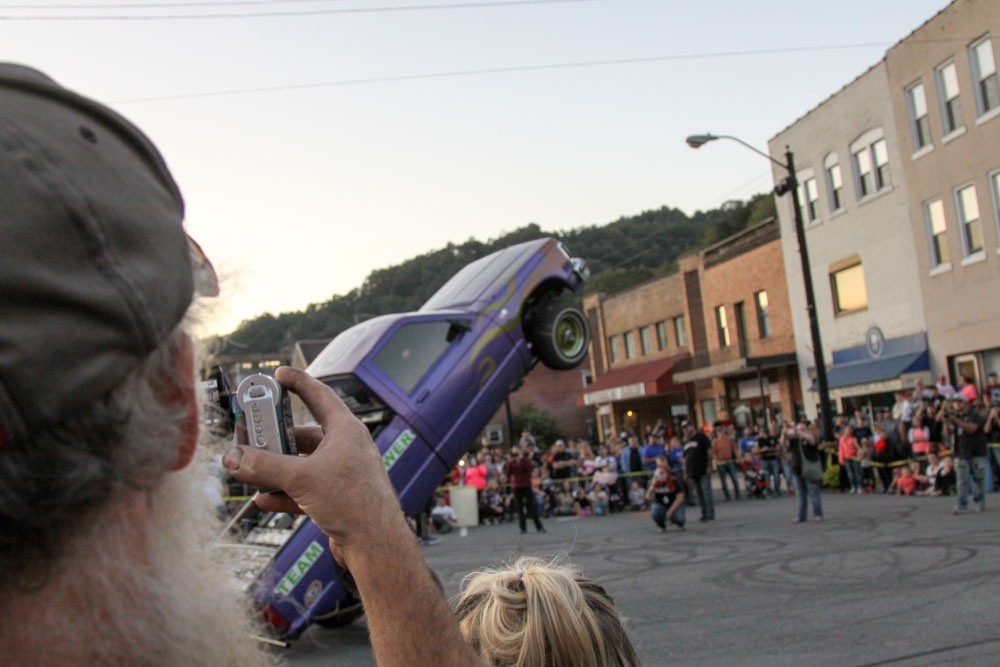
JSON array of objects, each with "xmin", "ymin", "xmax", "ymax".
[
  {"xmin": 976, "ymin": 501, "xmax": 987, "ymax": 514},
  {"xmin": 955, "ymin": 504, "xmax": 968, "ymax": 514},
  {"xmin": 793, "ymin": 515, "xmax": 805, "ymax": 524},
  {"xmin": 422, "ymin": 536, "xmax": 440, "ymax": 547}
]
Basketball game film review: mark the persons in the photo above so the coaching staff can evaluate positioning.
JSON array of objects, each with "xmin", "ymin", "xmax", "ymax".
[
  {"xmin": 0, "ymin": 61, "xmax": 483, "ymax": 666},
  {"xmin": 412, "ymin": 371, "xmax": 1000, "ymax": 547},
  {"xmin": 454, "ymin": 557, "xmax": 642, "ymax": 667}
]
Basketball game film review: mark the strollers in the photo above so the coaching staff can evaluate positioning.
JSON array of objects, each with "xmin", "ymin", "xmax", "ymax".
[{"xmin": 741, "ymin": 458, "xmax": 770, "ymax": 500}]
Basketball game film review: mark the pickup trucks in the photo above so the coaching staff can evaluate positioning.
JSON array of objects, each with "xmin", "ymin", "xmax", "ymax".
[{"xmin": 218, "ymin": 238, "xmax": 590, "ymax": 648}]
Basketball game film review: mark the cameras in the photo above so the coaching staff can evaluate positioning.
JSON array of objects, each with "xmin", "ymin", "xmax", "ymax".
[{"xmin": 201, "ymin": 372, "xmax": 302, "ymax": 494}]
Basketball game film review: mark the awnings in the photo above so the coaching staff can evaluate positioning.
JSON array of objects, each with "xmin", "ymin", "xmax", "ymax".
[
  {"xmin": 573, "ymin": 351, "xmax": 693, "ymax": 408},
  {"xmin": 806, "ymin": 331, "xmax": 935, "ymax": 400}
]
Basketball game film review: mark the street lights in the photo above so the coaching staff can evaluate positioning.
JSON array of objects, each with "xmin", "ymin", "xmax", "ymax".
[{"xmin": 686, "ymin": 132, "xmax": 838, "ymax": 472}]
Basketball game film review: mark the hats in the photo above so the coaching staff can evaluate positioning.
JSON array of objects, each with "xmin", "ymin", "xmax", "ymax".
[
  {"xmin": 952, "ymin": 394, "xmax": 967, "ymax": 402},
  {"xmin": 0, "ymin": 60, "xmax": 220, "ymax": 443},
  {"xmin": 680, "ymin": 420, "xmax": 692, "ymax": 429}
]
